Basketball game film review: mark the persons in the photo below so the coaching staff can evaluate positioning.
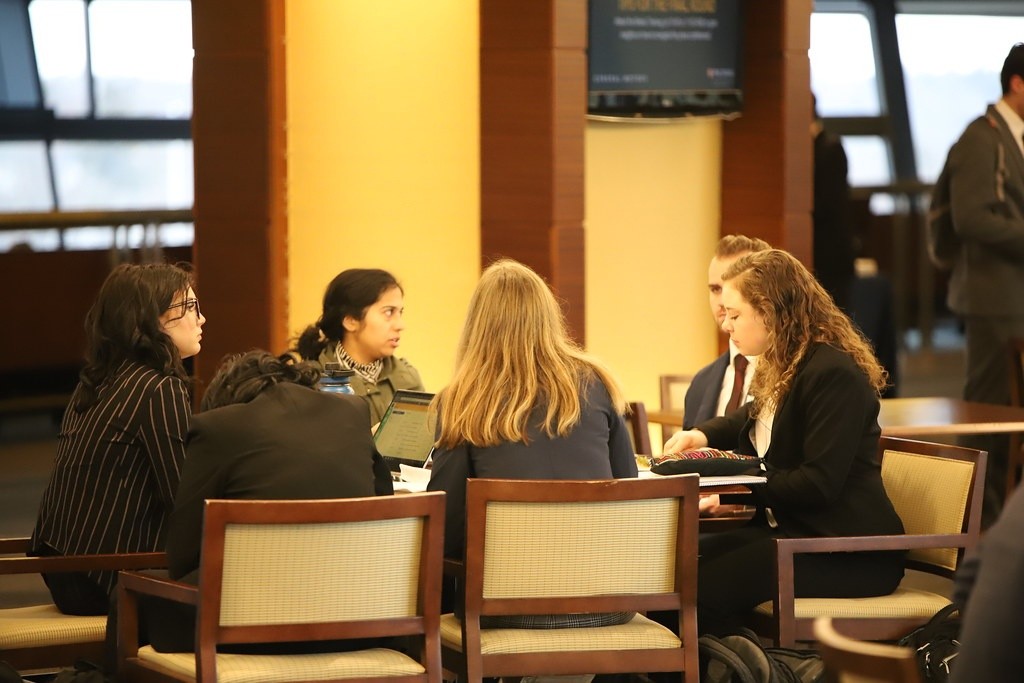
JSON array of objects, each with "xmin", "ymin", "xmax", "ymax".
[
  {"xmin": 101, "ymin": 350, "xmax": 394, "ymax": 683},
  {"xmin": 664, "ymin": 248, "xmax": 908, "ymax": 683},
  {"xmin": 948, "ymin": 45, "xmax": 1024, "ymax": 532},
  {"xmin": 947, "ymin": 469, "xmax": 1024, "ymax": 683},
  {"xmin": 26, "ymin": 263, "xmax": 206, "ymax": 616},
  {"xmin": 810, "ymin": 94, "xmax": 849, "ymax": 314},
  {"xmin": 295, "ymin": 269, "xmax": 426, "ymax": 436},
  {"xmin": 427, "ymin": 259, "xmax": 639, "ymax": 683},
  {"xmin": 682, "ymin": 235, "xmax": 773, "ymax": 518}
]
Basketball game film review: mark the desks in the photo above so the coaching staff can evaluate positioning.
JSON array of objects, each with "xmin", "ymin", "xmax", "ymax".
[{"xmin": 878, "ymin": 397, "xmax": 1024, "ymax": 437}]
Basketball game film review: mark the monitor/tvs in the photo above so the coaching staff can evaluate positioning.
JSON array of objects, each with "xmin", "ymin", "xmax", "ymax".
[{"xmin": 584, "ymin": 0, "xmax": 743, "ymax": 124}]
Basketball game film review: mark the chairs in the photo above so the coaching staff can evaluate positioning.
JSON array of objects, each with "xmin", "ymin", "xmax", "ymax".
[
  {"xmin": 754, "ymin": 437, "xmax": 988, "ymax": 683},
  {"xmin": 117, "ymin": 473, "xmax": 700, "ymax": 683},
  {"xmin": 0, "ymin": 536, "xmax": 166, "ymax": 683},
  {"xmin": 620, "ymin": 375, "xmax": 688, "ymax": 456}
]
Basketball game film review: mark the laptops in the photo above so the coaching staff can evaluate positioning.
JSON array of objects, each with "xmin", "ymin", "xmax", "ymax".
[{"xmin": 373, "ymin": 390, "xmax": 437, "ymax": 475}]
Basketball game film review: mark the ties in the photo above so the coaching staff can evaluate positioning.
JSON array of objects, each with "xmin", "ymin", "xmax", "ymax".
[{"xmin": 724, "ymin": 353, "xmax": 749, "ymax": 416}]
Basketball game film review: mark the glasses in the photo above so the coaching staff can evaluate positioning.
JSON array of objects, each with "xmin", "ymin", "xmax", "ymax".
[{"xmin": 166, "ymin": 297, "xmax": 201, "ymax": 320}]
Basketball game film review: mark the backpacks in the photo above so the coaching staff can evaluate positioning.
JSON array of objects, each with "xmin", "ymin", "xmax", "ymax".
[
  {"xmin": 927, "ymin": 115, "xmax": 1004, "ymax": 265},
  {"xmin": 698, "ymin": 627, "xmax": 824, "ymax": 683},
  {"xmin": 898, "ymin": 603, "xmax": 968, "ymax": 683}
]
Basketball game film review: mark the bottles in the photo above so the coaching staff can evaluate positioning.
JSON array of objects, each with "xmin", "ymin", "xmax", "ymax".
[{"xmin": 318, "ymin": 362, "xmax": 356, "ymax": 396}]
